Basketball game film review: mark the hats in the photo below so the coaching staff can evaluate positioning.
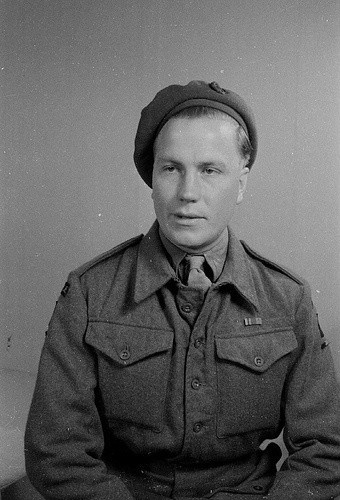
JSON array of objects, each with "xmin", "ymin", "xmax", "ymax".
[{"xmin": 132, "ymin": 80, "xmax": 257, "ymax": 189}]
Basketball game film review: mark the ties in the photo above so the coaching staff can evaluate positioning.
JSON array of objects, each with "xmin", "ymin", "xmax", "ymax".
[{"xmin": 185, "ymin": 254, "xmax": 212, "ymax": 297}]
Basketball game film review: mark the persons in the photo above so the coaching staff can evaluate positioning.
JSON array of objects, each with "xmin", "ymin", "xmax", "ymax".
[{"xmin": 24, "ymin": 78, "xmax": 340, "ymax": 500}]
